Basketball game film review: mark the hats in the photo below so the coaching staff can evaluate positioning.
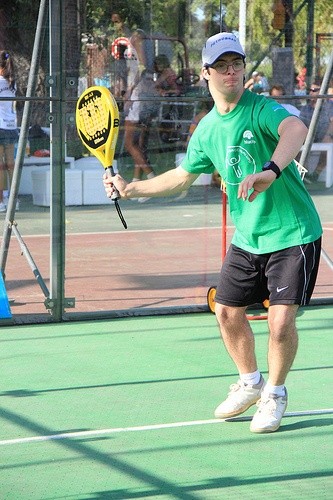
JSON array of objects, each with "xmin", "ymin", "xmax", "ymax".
[{"xmin": 202, "ymin": 33, "xmax": 245, "ymax": 67}]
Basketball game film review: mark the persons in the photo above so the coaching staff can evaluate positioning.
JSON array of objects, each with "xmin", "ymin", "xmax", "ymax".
[
  {"xmin": 78, "ymin": 6, "xmax": 333, "ymax": 203},
  {"xmin": 102, "ymin": 32, "xmax": 323, "ymax": 433},
  {"xmin": 0, "ymin": 49, "xmax": 20, "ymax": 212}
]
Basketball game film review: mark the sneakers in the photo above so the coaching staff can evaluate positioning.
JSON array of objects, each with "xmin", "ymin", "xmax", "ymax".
[
  {"xmin": 250, "ymin": 386, "xmax": 287, "ymax": 433},
  {"xmin": 131, "ymin": 197, "xmax": 151, "ymax": 202},
  {"xmin": 214, "ymin": 374, "xmax": 264, "ymax": 418},
  {"xmin": 0, "ymin": 202, "xmax": 7, "ymax": 213},
  {"xmin": 15, "ymin": 202, "xmax": 19, "ymax": 211}
]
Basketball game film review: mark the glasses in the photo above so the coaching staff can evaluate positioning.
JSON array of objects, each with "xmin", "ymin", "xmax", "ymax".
[{"xmin": 208, "ymin": 61, "xmax": 243, "ymax": 74}]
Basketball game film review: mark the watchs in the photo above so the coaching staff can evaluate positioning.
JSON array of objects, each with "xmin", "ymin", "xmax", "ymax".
[{"xmin": 262, "ymin": 160, "xmax": 282, "ymax": 180}]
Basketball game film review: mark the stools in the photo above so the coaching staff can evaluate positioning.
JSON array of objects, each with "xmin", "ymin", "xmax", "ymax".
[{"xmin": 174, "ymin": 152, "xmax": 212, "ymax": 186}]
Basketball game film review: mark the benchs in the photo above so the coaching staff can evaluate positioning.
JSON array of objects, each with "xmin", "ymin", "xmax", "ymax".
[
  {"xmin": 5, "ymin": 156, "xmax": 119, "ymax": 207},
  {"xmin": 300, "ymin": 142, "xmax": 333, "ymax": 188}
]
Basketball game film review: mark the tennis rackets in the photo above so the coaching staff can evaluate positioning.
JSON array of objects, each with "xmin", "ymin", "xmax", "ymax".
[{"xmin": 76, "ymin": 85, "xmax": 120, "ymax": 202}]
